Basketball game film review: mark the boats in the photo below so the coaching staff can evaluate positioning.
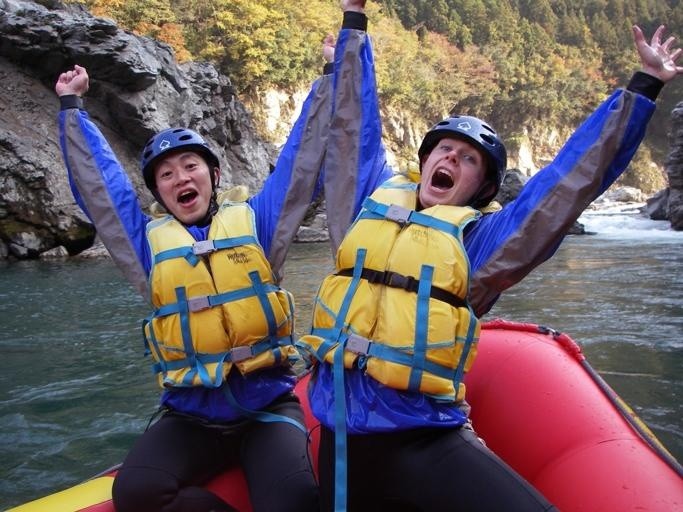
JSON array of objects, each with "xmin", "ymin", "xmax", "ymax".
[{"xmin": 1, "ymin": 314, "xmax": 681, "ymax": 512}]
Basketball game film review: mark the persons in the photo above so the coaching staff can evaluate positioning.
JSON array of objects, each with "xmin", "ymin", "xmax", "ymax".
[
  {"xmin": 294, "ymin": 1, "xmax": 683, "ymax": 510},
  {"xmin": 54, "ymin": 32, "xmax": 337, "ymax": 511}
]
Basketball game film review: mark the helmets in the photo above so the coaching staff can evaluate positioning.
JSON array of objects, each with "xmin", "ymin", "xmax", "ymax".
[
  {"xmin": 140, "ymin": 127, "xmax": 220, "ymax": 190},
  {"xmin": 418, "ymin": 115, "xmax": 507, "ymax": 208}
]
